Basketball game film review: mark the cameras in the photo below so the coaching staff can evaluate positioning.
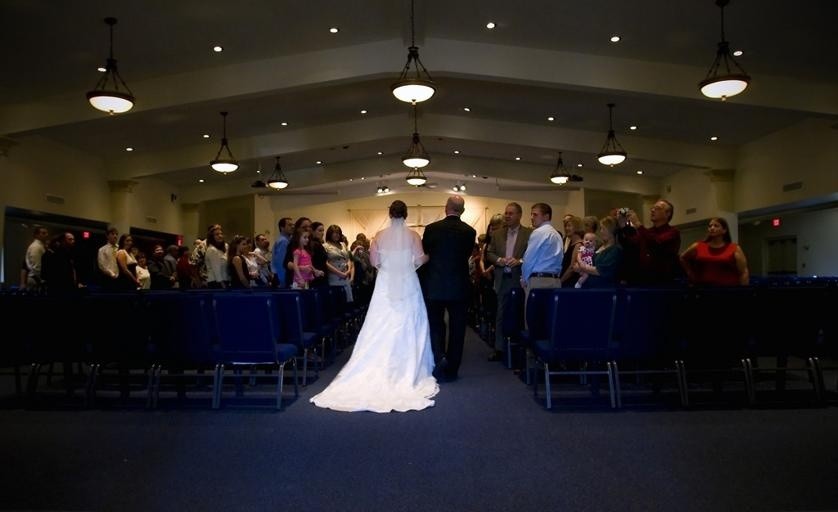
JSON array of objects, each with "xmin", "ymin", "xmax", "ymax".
[{"xmin": 619, "ymin": 208, "xmax": 629, "ymax": 214}]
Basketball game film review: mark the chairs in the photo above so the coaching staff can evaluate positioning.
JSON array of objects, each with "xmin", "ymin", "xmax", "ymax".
[
  {"xmin": 0, "ymin": 279, "xmax": 375, "ymax": 413},
  {"xmin": 468, "ymin": 273, "xmax": 838, "ymax": 412}
]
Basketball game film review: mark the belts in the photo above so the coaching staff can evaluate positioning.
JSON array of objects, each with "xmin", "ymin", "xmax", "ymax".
[{"xmin": 529, "ymin": 271, "xmax": 563, "ymax": 279}]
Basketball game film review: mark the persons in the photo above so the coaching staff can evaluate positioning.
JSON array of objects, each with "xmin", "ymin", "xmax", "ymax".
[
  {"xmin": 519, "ymin": 202, "xmax": 563, "ymax": 332},
  {"xmin": 367, "ymin": 199, "xmax": 430, "ymax": 393},
  {"xmin": 418, "ymin": 193, "xmax": 477, "ymax": 383},
  {"xmin": 484, "ymin": 201, "xmax": 531, "ymax": 364},
  {"xmin": 616, "ymin": 199, "xmax": 680, "ymax": 283}
]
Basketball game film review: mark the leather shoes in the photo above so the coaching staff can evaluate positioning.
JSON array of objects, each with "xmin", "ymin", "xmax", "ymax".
[
  {"xmin": 439, "ymin": 356, "xmax": 458, "ymax": 381},
  {"xmin": 488, "ymin": 350, "xmax": 504, "ymax": 361}
]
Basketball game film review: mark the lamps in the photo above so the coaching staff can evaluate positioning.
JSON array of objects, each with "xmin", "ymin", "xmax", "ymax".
[
  {"xmin": 83, "ymin": 15, "xmax": 137, "ymax": 116},
  {"xmin": 267, "ymin": 157, "xmax": 289, "ymax": 189},
  {"xmin": 699, "ymin": 1, "xmax": 752, "ymax": 102},
  {"xmin": 208, "ymin": 111, "xmax": 241, "ymax": 175},
  {"xmin": 402, "ymin": 106, "xmax": 430, "ymax": 169},
  {"xmin": 390, "ymin": 1, "xmax": 436, "ymax": 105},
  {"xmin": 550, "ymin": 150, "xmax": 571, "ymax": 186},
  {"xmin": 404, "ymin": 166, "xmax": 428, "ymax": 186},
  {"xmin": 596, "ymin": 101, "xmax": 627, "ymax": 167}
]
